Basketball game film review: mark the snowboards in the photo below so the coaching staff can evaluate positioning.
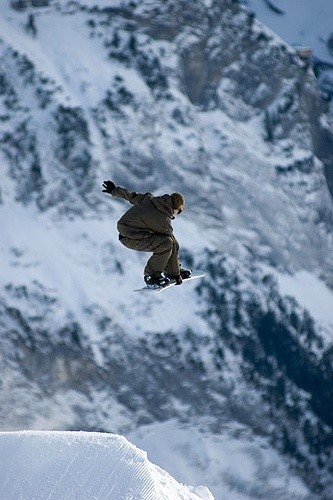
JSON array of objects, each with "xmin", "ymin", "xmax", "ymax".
[{"xmin": 132, "ymin": 269, "xmax": 205, "ymax": 293}]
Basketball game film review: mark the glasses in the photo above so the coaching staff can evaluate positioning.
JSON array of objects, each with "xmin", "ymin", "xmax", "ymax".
[{"xmin": 175, "ymin": 207, "xmax": 183, "ymax": 214}]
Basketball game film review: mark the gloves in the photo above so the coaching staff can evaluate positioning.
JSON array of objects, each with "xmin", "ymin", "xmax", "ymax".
[
  {"xmin": 174, "ymin": 275, "xmax": 183, "ymax": 285},
  {"xmin": 102, "ymin": 180, "xmax": 115, "ymax": 194}
]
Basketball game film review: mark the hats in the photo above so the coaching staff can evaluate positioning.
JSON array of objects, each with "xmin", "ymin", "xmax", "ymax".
[{"xmin": 171, "ymin": 193, "xmax": 183, "ymax": 209}]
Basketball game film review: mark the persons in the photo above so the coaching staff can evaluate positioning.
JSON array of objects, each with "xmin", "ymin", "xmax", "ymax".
[{"xmin": 101, "ymin": 179, "xmax": 190, "ymax": 287}]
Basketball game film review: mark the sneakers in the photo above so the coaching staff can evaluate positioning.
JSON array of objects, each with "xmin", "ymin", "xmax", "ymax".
[
  {"xmin": 164, "ymin": 269, "xmax": 193, "ymax": 279},
  {"xmin": 144, "ymin": 273, "xmax": 170, "ymax": 287}
]
